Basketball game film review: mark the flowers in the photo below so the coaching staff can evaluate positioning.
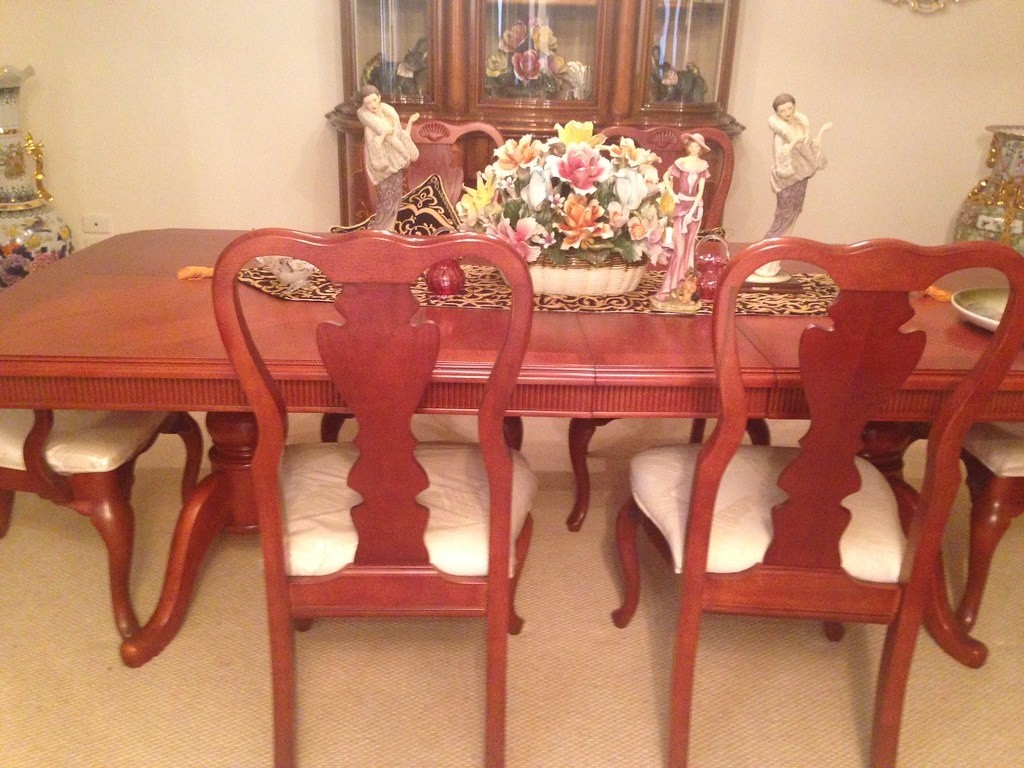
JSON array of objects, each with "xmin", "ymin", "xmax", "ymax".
[
  {"xmin": 456, "ymin": 120, "xmax": 671, "ymax": 267},
  {"xmin": 484, "ymin": 18, "xmax": 568, "ymax": 99}
]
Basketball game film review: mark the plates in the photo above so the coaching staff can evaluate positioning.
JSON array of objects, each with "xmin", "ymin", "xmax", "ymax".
[{"xmin": 950, "ymin": 284, "xmax": 1010, "ymax": 332}]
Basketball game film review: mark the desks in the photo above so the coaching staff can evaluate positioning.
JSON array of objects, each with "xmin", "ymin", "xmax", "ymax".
[{"xmin": 1, "ymin": 228, "xmax": 1024, "ymax": 669}]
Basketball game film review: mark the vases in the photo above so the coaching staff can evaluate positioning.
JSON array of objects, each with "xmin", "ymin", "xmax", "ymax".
[
  {"xmin": 0, "ymin": 64, "xmax": 75, "ymax": 290},
  {"xmin": 953, "ymin": 125, "xmax": 1024, "ymax": 256},
  {"xmin": 529, "ymin": 249, "xmax": 648, "ymax": 296}
]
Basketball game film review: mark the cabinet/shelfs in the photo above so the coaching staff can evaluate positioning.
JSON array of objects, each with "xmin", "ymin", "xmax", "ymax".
[{"xmin": 325, "ymin": 0, "xmax": 746, "ymax": 227}]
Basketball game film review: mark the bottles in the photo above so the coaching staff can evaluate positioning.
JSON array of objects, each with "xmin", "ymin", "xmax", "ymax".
[{"xmin": 952, "ymin": 123, "xmax": 1024, "ymax": 258}]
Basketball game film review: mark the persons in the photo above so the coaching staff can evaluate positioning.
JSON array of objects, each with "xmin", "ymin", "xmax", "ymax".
[
  {"xmin": 357, "ymin": 84, "xmax": 421, "ymax": 231},
  {"xmin": 660, "ymin": 132, "xmax": 711, "ymax": 300},
  {"xmin": 754, "ymin": 94, "xmax": 832, "ymax": 276}
]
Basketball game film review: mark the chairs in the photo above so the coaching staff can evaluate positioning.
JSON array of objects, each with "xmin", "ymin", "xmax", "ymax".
[
  {"xmin": 611, "ymin": 238, "xmax": 1024, "ymax": 768},
  {"xmin": 211, "ymin": 228, "xmax": 539, "ymax": 768},
  {"xmin": 956, "ymin": 422, "xmax": 1024, "ymax": 632},
  {"xmin": 0, "ymin": 408, "xmax": 204, "ymax": 640},
  {"xmin": 566, "ymin": 127, "xmax": 770, "ymax": 531},
  {"xmin": 320, "ymin": 118, "xmax": 524, "ymax": 451}
]
